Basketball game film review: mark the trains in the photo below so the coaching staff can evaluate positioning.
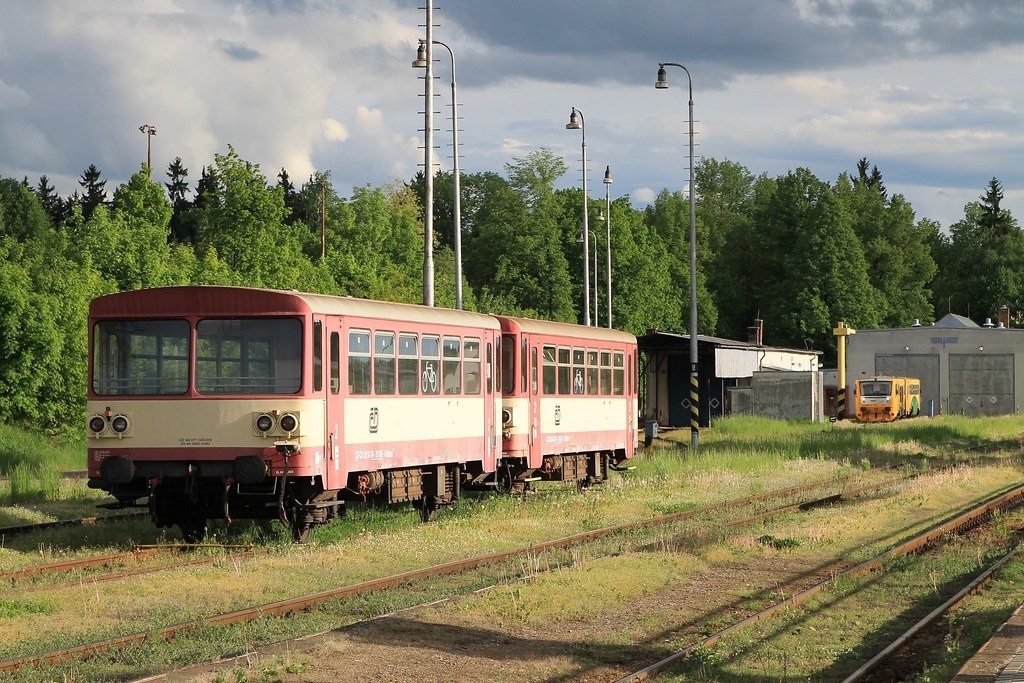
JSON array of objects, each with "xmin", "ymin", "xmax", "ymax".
[{"xmin": 83, "ymin": 284, "xmax": 645, "ymax": 540}]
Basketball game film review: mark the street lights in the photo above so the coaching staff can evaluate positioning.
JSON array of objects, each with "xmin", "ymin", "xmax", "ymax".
[
  {"xmin": 653, "ymin": 61, "xmax": 701, "ymax": 455},
  {"xmin": 565, "ymin": 106, "xmax": 591, "ymax": 325},
  {"xmin": 575, "ymin": 227, "xmax": 598, "ymax": 327},
  {"xmin": 603, "ymin": 165, "xmax": 614, "ymax": 327},
  {"xmin": 408, "ymin": 36, "xmax": 468, "ymax": 309},
  {"xmin": 139, "ymin": 123, "xmax": 158, "ymax": 178}
]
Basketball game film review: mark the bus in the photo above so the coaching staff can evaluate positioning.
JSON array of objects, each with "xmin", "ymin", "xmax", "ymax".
[{"xmin": 853, "ymin": 375, "xmax": 921, "ymax": 424}]
